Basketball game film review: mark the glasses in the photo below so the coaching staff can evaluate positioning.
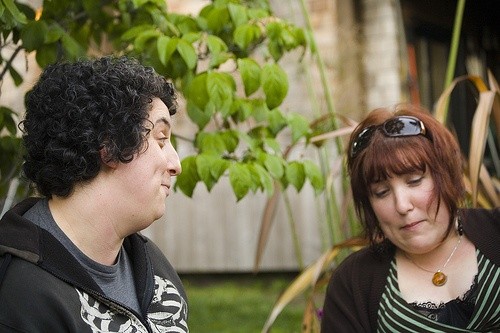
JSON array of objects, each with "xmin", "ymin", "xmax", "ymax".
[{"xmin": 350, "ymin": 116, "xmax": 431, "ymax": 162}]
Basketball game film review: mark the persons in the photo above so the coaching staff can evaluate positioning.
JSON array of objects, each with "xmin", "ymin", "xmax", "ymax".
[
  {"xmin": 0, "ymin": 56, "xmax": 190, "ymax": 333},
  {"xmin": 321, "ymin": 106, "xmax": 499, "ymax": 333}
]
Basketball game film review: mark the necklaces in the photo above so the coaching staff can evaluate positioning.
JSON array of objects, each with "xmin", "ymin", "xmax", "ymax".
[{"xmin": 403, "ymin": 233, "xmax": 463, "ymax": 287}]
